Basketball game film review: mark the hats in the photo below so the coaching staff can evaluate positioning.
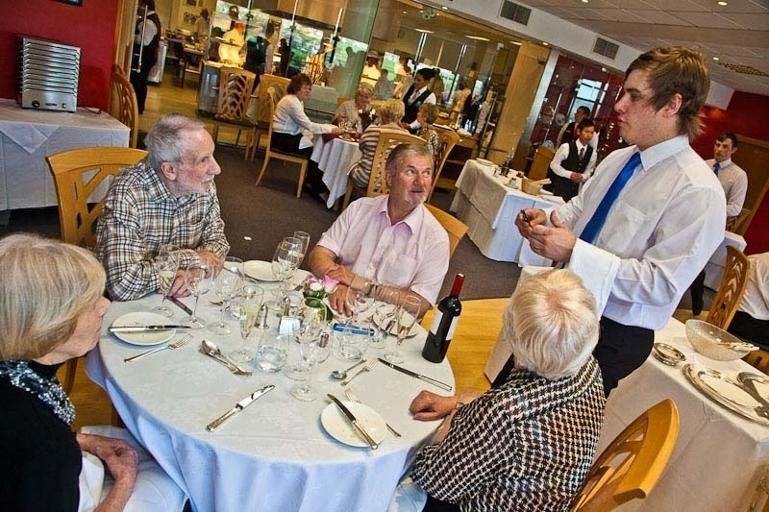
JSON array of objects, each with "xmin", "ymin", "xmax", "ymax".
[{"xmin": 366, "ymin": 50, "xmax": 381, "ymax": 60}]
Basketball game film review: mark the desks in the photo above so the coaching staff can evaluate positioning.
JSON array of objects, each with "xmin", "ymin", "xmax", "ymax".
[
  {"xmin": 306, "ymin": 84, "xmax": 338, "ymax": 123},
  {"xmin": 0, "ymin": 95, "xmax": 131, "ymax": 225},
  {"xmin": 147, "ymin": 40, "xmax": 168, "ymax": 84},
  {"xmin": 177, "ymin": 43, "xmax": 205, "ymax": 88},
  {"xmin": 448, "ymin": 159, "xmax": 566, "ymax": 267},
  {"xmin": 703, "ymin": 230, "xmax": 748, "ymax": 292}
]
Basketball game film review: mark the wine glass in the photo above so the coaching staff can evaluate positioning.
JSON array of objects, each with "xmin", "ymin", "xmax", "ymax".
[{"xmin": 152, "ymin": 231, "xmax": 423, "ymax": 401}]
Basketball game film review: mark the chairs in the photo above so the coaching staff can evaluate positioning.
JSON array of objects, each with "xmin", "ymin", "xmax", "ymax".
[
  {"xmin": 254, "ymin": 87, "xmax": 307, "ymax": 200},
  {"xmin": 421, "ymin": 203, "xmax": 470, "ymax": 260},
  {"xmin": 341, "ymin": 130, "xmax": 427, "ymax": 210},
  {"xmin": 116, "ymin": 62, "xmax": 126, "ymax": 76},
  {"xmin": 250, "ymin": 73, "xmax": 291, "ymax": 162},
  {"xmin": 705, "ymin": 244, "xmax": 750, "ymax": 331},
  {"xmin": 428, "ymin": 130, "xmax": 460, "ymax": 203},
  {"xmin": 566, "ymin": 397, "xmax": 679, "ymax": 512},
  {"xmin": 211, "ymin": 66, "xmax": 253, "ymax": 162},
  {"xmin": 110, "ymin": 73, "xmax": 140, "ymax": 149},
  {"xmin": 46, "ymin": 148, "xmax": 148, "ymax": 428}
]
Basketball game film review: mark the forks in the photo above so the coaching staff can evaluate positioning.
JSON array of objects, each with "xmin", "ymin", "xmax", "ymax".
[
  {"xmin": 344, "ymin": 388, "xmax": 402, "ymax": 439},
  {"xmin": 125, "ymin": 333, "xmax": 193, "ymax": 364}
]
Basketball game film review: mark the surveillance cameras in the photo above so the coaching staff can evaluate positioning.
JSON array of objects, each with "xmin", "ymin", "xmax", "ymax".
[{"xmin": 422, "ymin": 7, "xmax": 433, "ymax": 20}]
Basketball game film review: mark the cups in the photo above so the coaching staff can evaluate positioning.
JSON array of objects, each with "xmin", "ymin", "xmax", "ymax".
[
  {"xmin": 509, "ymin": 178, "xmax": 516, "ymax": 187},
  {"xmin": 336, "ymin": 116, "xmax": 365, "ymax": 140},
  {"xmin": 501, "ymin": 166, "xmax": 509, "ymax": 177},
  {"xmin": 493, "ymin": 167, "xmax": 500, "ymax": 177}
]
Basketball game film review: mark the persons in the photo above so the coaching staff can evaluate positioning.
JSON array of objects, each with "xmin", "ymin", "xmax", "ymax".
[
  {"xmin": 0, "ymin": 232, "xmax": 191, "ymax": 512},
  {"xmin": 549, "ymin": 119, "xmax": 597, "ymax": 203},
  {"xmin": 409, "ymin": 267, "xmax": 606, "ymax": 512},
  {"xmin": 490, "ymin": 46, "xmax": 727, "ymax": 399},
  {"xmin": 727, "ymin": 251, "xmax": 769, "ymax": 350},
  {"xmin": 557, "ymin": 106, "xmax": 598, "ymax": 153},
  {"xmin": 131, "ymin": 0, "xmax": 161, "ymax": 115},
  {"xmin": 690, "ymin": 133, "xmax": 748, "ymax": 318},
  {"xmin": 308, "ymin": 142, "xmax": 450, "ymax": 318},
  {"xmin": 94, "ymin": 113, "xmax": 230, "ymax": 302}
]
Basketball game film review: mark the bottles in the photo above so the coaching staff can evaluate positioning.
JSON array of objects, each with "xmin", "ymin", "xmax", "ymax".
[{"xmin": 420, "ymin": 272, "xmax": 466, "ymax": 363}]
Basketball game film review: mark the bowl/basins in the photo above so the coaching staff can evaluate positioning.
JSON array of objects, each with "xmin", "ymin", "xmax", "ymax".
[
  {"xmin": 434, "ymin": 115, "xmax": 451, "ymax": 125},
  {"xmin": 652, "ymin": 343, "xmax": 686, "ymax": 366},
  {"xmin": 685, "ymin": 318, "xmax": 750, "ymax": 361},
  {"xmin": 521, "ymin": 178, "xmax": 544, "ymax": 195}
]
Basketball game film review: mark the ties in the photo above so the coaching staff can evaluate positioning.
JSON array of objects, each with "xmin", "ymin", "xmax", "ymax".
[
  {"xmin": 574, "ymin": 124, "xmax": 580, "ymax": 139},
  {"xmin": 579, "ymin": 148, "xmax": 585, "ymax": 162},
  {"xmin": 412, "ymin": 90, "xmax": 420, "ymax": 102},
  {"xmin": 712, "ymin": 163, "xmax": 720, "ymax": 178},
  {"xmin": 579, "ymin": 153, "xmax": 641, "ymax": 245}
]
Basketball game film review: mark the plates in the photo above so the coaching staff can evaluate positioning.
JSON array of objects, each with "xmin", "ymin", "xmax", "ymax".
[
  {"xmin": 318, "ymin": 400, "xmax": 390, "ymax": 447},
  {"xmin": 683, "ymin": 362, "xmax": 769, "ymax": 424},
  {"xmin": 476, "ymin": 157, "xmax": 494, "ymax": 165},
  {"xmin": 505, "ymin": 182, "xmax": 519, "ymax": 190},
  {"xmin": 111, "ymin": 312, "xmax": 175, "ymax": 346}
]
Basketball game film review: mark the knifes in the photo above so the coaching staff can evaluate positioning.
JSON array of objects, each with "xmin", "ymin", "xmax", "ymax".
[
  {"xmin": 376, "ymin": 355, "xmax": 457, "ymax": 392},
  {"xmin": 206, "ymin": 384, "xmax": 276, "ymax": 432},
  {"xmin": 326, "ymin": 393, "xmax": 379, "ymax": 450},
  {"xmin": 107, "ymin": 323, "xmax": 193, "ymax": 335}
]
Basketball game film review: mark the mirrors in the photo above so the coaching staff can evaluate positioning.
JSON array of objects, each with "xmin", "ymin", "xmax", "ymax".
[{"xmin": 477, "ymin": 96, "xmax": 507, "ymax": 160}]
